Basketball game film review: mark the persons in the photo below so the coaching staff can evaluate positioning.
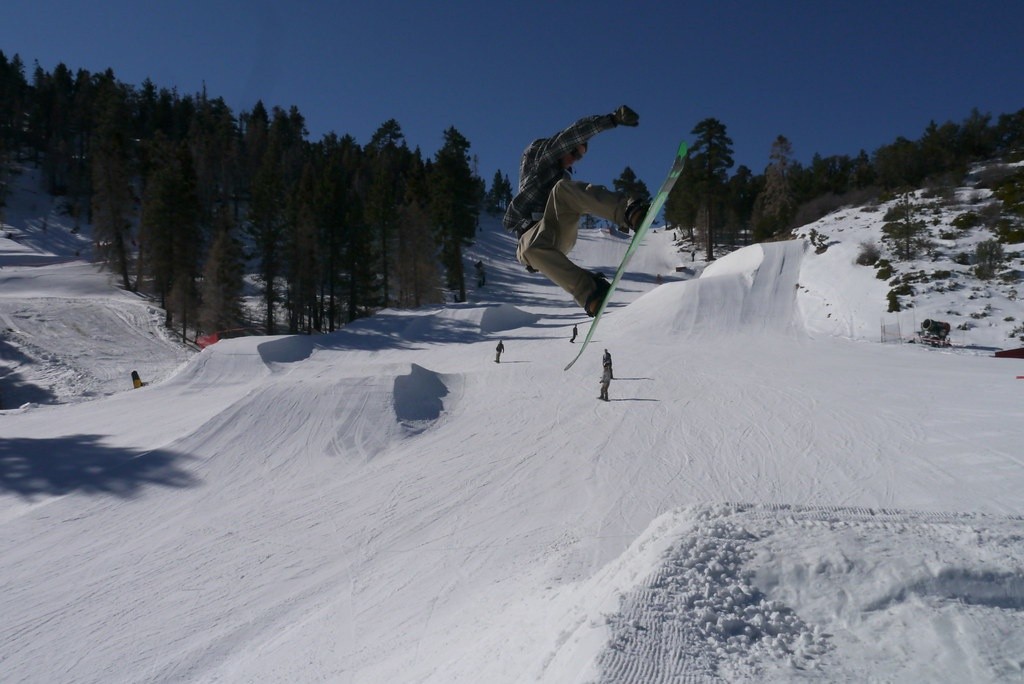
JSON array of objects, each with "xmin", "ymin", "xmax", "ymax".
[
  {"xmin": 599, "ymin": 363, "xmax": 611, "ymax": 400},
  {"xmin": 570, "ymin": 324, "xmax": 577, "ymax": 342},
  {"xmin": 504, "ymin": 104, "xmax": 651, "ymax": 316},
  {"xmin": 495, "ymin": 340, "xmax": 504, "ymax": 362},
  {"xmin": 603, "ymin": 349, "xmax": 613, "ymax": 379}
]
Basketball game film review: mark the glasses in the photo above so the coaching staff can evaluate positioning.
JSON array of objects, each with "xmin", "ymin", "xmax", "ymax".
[{"xmin": 571, "ymin": 148, "xmax": 583, "ymax": 160}]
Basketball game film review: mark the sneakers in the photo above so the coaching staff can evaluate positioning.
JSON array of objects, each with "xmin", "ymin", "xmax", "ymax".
[
  {"xmin": 584, "ymin": 270, "xmax": 611, "ymax": 318},
  {"xmin": 626, "ymin": 196, "xmax": 650, "ymax": 231}
]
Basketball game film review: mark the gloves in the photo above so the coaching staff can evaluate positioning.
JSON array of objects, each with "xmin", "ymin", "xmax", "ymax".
[{"xmin": 614, "ymin": 105, "xmax": 640, "ymax": 127}]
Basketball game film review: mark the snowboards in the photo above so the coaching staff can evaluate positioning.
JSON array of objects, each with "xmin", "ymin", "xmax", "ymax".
[
  {"xmin": 596, "ymin": 396, "xmax": 611, "ymax": 402},
  {"xmin": 564, "ymin": 141, "xmax": 688, "ymax": 371}
]
